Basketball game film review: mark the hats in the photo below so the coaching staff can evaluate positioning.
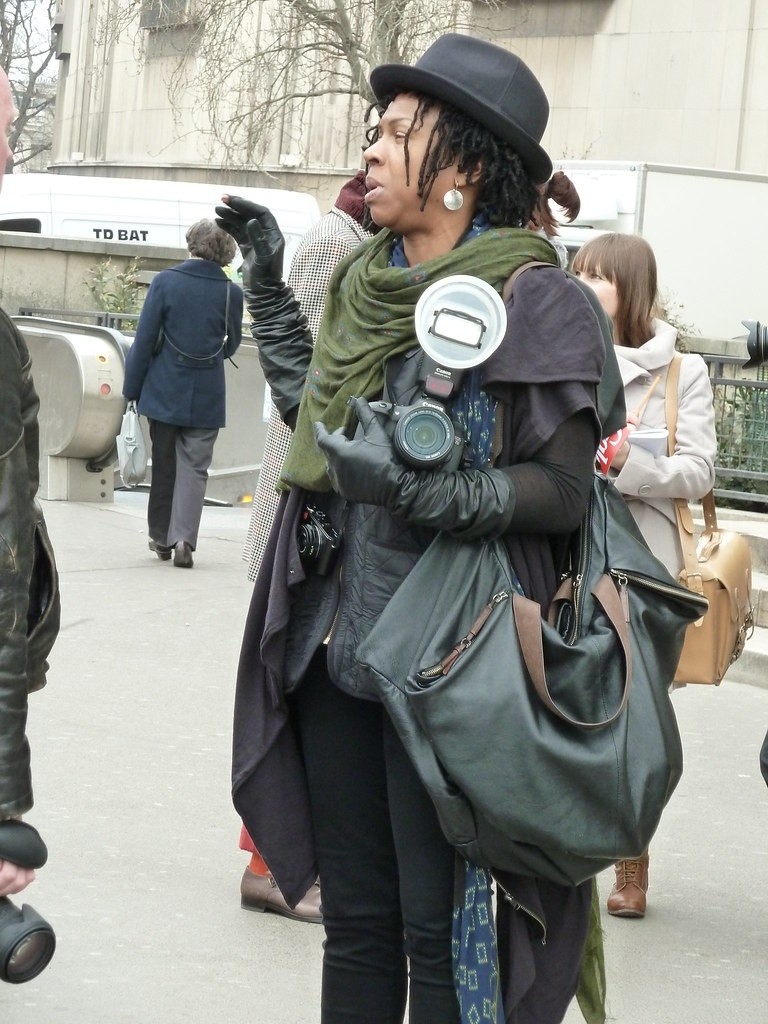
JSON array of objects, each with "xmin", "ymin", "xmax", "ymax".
[
  {"xmin": 370, "ymin": 34, "xmax": 555, "ymax": 183},
  {"xmin": 335, "ymin": 171, "xmax": 369, "ymax": 223}
]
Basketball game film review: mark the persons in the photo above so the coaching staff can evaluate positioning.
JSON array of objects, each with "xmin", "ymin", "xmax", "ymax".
[
  {"xmin": 570, "ymin": 233, "xmax": 718, "ymax": 917},
  {"xmin": 121, "ymin": 218, "xmax": 243, "ymax": 568},
  {"xmin": 0, "ymin": 311, "xmax": 63, "ymax": 893},
  {"xmin": 215, "ymin": 35, "xmax": 628, "ymax": 1023},
  {"xmin": 238, "ymin": 168, "xmax": 384, "ymax": 923}
]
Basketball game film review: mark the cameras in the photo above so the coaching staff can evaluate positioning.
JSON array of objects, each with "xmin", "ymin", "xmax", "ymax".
[
  {"xmin": 297, "ymin": 503, "xmax": 342, "ymax": 576},
  {"xmin": 354, "ymin": 274, "xmax": 507, "ymax": 472}
]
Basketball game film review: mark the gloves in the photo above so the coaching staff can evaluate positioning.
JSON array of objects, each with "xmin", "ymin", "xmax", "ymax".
[
  {"xmin": 213, "ymin": 194, "xmax": 316, "ymax": 422},
  {"xmin": 314, "ymin": 398, "xmax": 518, "ymax": 542}
]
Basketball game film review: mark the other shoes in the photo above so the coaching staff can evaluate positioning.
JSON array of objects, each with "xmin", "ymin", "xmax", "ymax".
[
  {"xmin": 173, "ymin": 542, "xmax": 194, "ymax": 568},
  {"xmin": 148, "ymin": 539, "xmax": 173, "ymax": 560}
]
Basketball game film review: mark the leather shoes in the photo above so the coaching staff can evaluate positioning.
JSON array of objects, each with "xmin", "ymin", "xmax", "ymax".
[
  {"xmin": 241, "ymin": 865, "xmax": 323, "ymax": 923},
  {"xmin": 608, "ymin": 857, "xmax": 648, "ymax": 916}
]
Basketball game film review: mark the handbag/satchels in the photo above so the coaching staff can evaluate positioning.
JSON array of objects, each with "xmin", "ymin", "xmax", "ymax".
[
  {"xmin": 116, "ymin": 399, "xmax": 147, "ymax": 489},
  {"xmin": 664, "ymin": 349, "xmax": 754, "ymax": 686},
  {"xmin": 355, "ymin": 261, "xmax": 684, "ymax": 886}
]
